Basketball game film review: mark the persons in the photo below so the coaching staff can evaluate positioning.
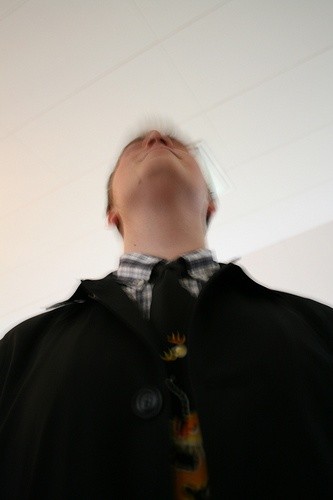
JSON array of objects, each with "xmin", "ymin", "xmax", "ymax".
[{"xmin": 1, "ymin": 128, "xmax": 332, "ymax": 499}]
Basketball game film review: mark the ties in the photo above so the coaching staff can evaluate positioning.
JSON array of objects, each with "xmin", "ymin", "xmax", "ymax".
[{"xmin": 145, "ymin": 255, "xmax": 200, "ymax": 362}]
